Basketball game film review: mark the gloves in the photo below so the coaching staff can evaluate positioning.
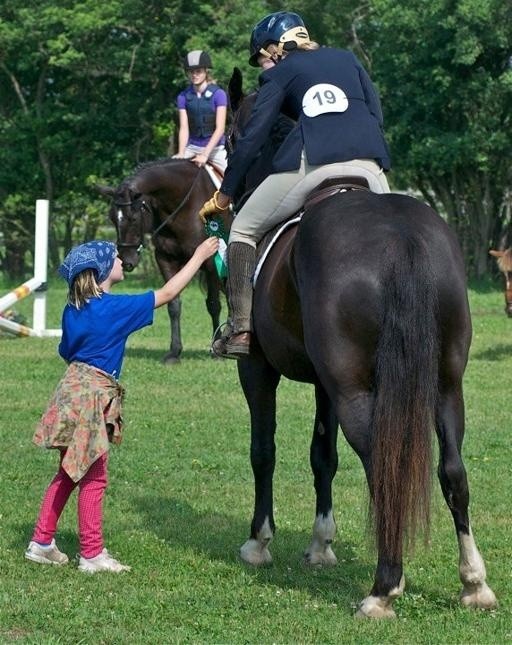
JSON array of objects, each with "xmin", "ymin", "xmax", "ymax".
[{"xmin": 198, "ymin": 191, "xmax": 231, "ymax": 223}]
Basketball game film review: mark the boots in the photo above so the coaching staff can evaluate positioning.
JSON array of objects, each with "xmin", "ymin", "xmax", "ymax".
[{"xmin": 211, "ymin": 242, "xmax": 256, "ymax": 359}]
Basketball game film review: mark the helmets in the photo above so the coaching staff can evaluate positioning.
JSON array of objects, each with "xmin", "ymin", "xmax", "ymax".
[
  {"xmin": 183, "ymin": 50, "xmax": 212, "ymax": 70},
  {"xmin": 248, "ymin": 11, "xmax": 309, "ymax": 67}
]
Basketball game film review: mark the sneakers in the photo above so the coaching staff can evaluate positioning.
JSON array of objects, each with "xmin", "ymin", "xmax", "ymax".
[
  {"xmin": 25, "ymin": 538, "xmax": 68, "ymax": 565},
  {"xmin": 78, "ymin": 548, "xmax": 132, "ymax": 574}
]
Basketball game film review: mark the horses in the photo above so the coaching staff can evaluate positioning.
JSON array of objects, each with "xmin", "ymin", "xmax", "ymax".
[
  {"xmin": 90, "ymin": 154, "xmax": 237, "ymax": 363},
  {"xmin": 222, "ymin": 64, "xmax": 498, "ymax": 621}
]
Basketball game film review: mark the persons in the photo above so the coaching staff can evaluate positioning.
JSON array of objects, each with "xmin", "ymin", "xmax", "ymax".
[
  {"xmin": 24, "ymin": 235, "xmax": 221, "ymax": 573},
  {"xmin": 197, "ymin": 11, "xmax": 392, "ymax": 359},
  {"xmin": 170, "ymin": 50, "xmax": 230, "ymax": 178}
]
